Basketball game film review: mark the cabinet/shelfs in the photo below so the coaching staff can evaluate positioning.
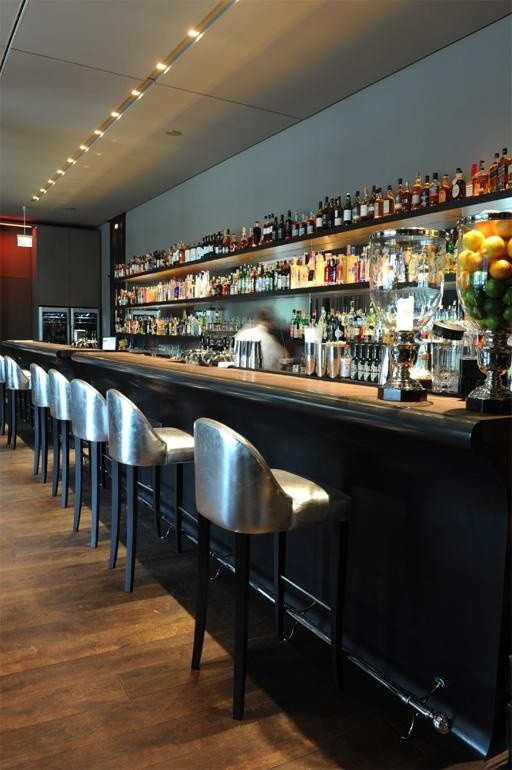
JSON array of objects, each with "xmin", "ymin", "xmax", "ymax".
[{"xmin": 107, "ymin": 179, "xmax": 512, "ymax": 398}]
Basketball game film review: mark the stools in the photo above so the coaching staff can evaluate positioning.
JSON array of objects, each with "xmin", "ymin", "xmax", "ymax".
[
  {"xmin": 69, "ymin": 378, "xmax": 163, "ymax": 549},
  {"xmin": 105, "ymin": 387, "xmax": 196, "ymax": 595},
  {"xmin": 1, "ymin": 353, "xmax": 72, "ymax": 509},
  {"xmin": 189, "ymin": 416, "xmax": 350, "ymax": 722}
]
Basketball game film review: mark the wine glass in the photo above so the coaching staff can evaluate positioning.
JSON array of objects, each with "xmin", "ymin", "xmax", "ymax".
[
  {"xmin": 363, "ymin": 223, "xmax": 456, "ymax": 402},
  {"xmin": 453, "ymin": 212, "xmax": 512, "ymax": 417}
]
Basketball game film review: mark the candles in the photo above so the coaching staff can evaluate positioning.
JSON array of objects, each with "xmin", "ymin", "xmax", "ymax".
[{"xmin": 392, "ymin": 295, "xmax": 417, "ymax": 334}]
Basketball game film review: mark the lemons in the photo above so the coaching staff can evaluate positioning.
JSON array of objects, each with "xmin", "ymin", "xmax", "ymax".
[{"xmin": 456, "ymin": 219, "xmax": 512, "ymax": 329}]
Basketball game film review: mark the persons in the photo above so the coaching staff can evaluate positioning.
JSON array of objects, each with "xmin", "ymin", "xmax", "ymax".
[{"xmin": 235, "ymin": 307, "xmax": 290, "ymax": 371}]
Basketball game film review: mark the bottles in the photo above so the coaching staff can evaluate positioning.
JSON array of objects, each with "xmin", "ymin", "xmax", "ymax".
[{"xmin": 112, "ymin": 147, "xmax": 512, "ymax": 391}]
